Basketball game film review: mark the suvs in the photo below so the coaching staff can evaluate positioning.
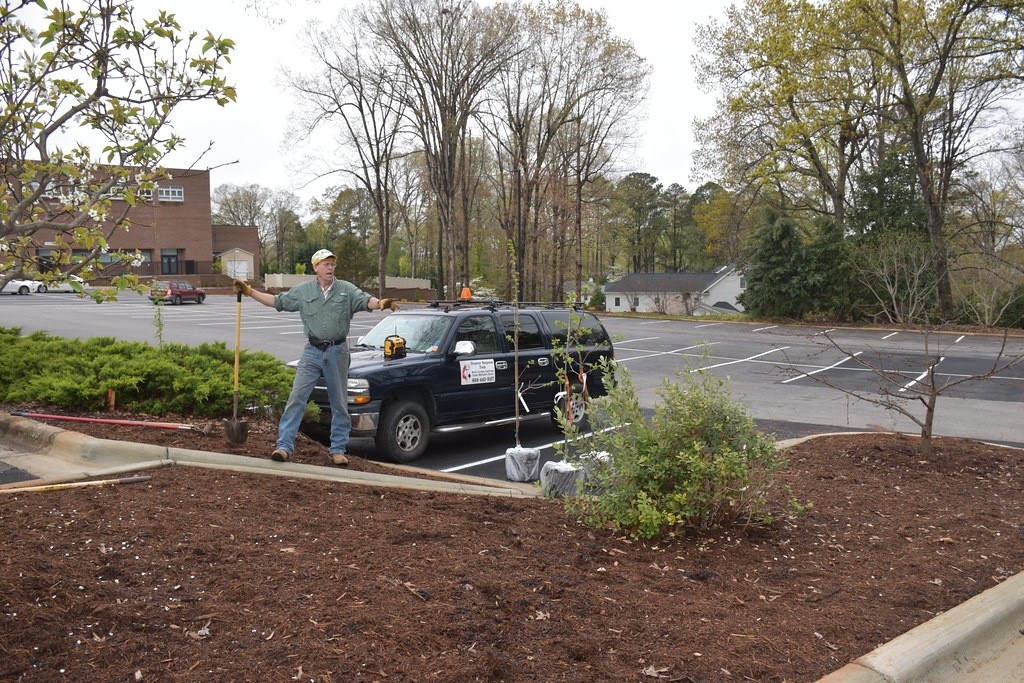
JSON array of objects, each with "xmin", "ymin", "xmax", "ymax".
[
  {"xmin": 147, "ymin": 280, "xmax": 207, "ymax": 306},
  {"xmin": 271, "ymin": 286, "xmax": 621, "ymax": 465}
]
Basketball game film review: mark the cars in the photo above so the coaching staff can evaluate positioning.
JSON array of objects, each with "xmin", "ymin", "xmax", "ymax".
[
  {"xmin": 0, "ymin": 275, "xmax": 36, "ymax": 295},
  {"xmin": 33, "ymin": 273, "xmax": 89, "ymax": 294}
]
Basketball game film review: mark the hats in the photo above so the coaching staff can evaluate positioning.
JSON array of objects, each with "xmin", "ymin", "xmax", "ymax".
[{"xmin": 311, "ymin": 249, "xmax": 338, "ymax": 265}]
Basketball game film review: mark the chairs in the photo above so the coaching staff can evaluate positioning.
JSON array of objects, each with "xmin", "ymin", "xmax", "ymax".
[{"xmin": 471, "ymin": 330, "xmax": 494, "ymax": 353}]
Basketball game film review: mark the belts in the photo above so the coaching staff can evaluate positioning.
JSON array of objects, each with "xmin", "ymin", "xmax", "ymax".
[{"xmin": 309, "ymin": 336, "xmax": 346, "ymax": 352}]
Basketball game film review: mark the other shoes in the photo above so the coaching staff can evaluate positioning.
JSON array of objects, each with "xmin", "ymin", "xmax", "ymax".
[
  {"xmin": 333, "ymin": 453, "xmax": 348, "ymax": 465},
  {"xmin": 272, "ymin": 449, "xmax": 288, "ymax": 462}
]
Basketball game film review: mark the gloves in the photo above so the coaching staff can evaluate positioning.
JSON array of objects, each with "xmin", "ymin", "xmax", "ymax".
[
  {"xmin": 233, "ymin": 279, "xmax": 251, "ymax": 297},
  {"xmin": 378, "ymin": 298, "xmax": 400, "ymax": 312}
]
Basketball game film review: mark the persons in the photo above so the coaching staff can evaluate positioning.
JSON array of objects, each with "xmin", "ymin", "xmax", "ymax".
[{"xmin": 233, "ymin": 248, "xmax": 400, "ymax": 466}]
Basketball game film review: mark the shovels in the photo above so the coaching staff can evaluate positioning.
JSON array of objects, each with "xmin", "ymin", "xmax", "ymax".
[{"xmin": 223, "ymin": 289, "xmax": 248, "ymax": 444}]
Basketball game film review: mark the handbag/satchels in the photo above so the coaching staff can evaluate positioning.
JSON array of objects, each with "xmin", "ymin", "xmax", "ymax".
[{"xmin": 384, "ymin": 335, "xmax": 407, "ymax": 360}]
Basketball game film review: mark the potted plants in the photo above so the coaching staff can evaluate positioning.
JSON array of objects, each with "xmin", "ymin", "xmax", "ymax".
[
  {"xmin": 573, "ymin": 276, "xmax": 625, "ymax": 487},
  {"xmin": 539, "ymin": 290, "xmax": 590, "ymax": 497},
  {"xmin": 506, "ymin": 239, "xmax": 540, "ymax": 481}
]
